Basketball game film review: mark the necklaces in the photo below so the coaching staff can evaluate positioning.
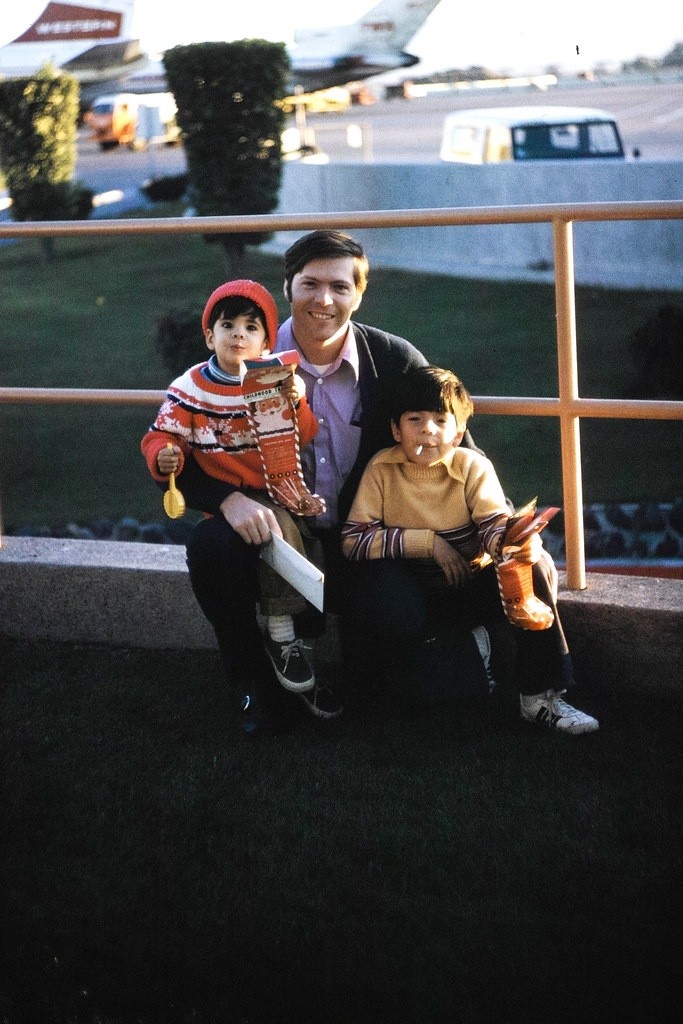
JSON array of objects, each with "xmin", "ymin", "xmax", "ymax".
[{"xmin": 291, "ymin": 329, "xmax": 309, "ymax": 362}]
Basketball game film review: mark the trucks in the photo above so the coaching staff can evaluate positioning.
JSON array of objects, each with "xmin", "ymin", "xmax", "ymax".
[{"xmin": 89, "ymin": 91, "xmax": 184, "ymax": 153}]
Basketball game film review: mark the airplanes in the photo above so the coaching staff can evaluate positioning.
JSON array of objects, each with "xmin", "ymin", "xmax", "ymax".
[{"xmin": 0, "ymin": 1, "xmax": 441, "ymax": 97}]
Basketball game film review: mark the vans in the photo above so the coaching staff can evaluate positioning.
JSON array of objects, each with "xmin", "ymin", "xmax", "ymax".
[{"xmin": 438, "ymin": 105, "xmax": 641, "ymax": 165}]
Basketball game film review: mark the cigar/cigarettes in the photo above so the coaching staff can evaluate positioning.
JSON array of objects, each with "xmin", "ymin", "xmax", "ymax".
[{"xmin": 415, "ymin": 444, "xmax": 424, "ymax": 455}]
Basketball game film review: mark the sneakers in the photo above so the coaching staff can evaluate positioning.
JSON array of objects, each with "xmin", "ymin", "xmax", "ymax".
[
  {"xmin": 518, "ymin": 688, "xmax": 598, "ymax": 735},
  {"xmin": 269, "ymin": 639, "xmax": 314, "ymax": 692},
  {"xmin": 464, "ymin": 627, "xmax": 491, "ymax": 698},
  {"xmin": 301, "ymin": 671, "xmax": 343, "ymax": 719}
]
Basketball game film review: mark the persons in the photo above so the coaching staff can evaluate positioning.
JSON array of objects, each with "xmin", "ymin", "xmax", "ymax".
[
  {"xmin": 186, "ymin": 228, "xmax": 515, "ymax": 742},
  {"xmin": 140, "ymin": 280, "xmax": 343, "ymax": 719},
  {"xmin": 344, "ymin": 365, "xmax": 599, "ymax": 735}
]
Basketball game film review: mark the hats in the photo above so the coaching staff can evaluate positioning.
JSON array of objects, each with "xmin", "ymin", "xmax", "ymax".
[{"xmin": 201, "ymin": 280, "xmax": 278, "ymax": 352}]
